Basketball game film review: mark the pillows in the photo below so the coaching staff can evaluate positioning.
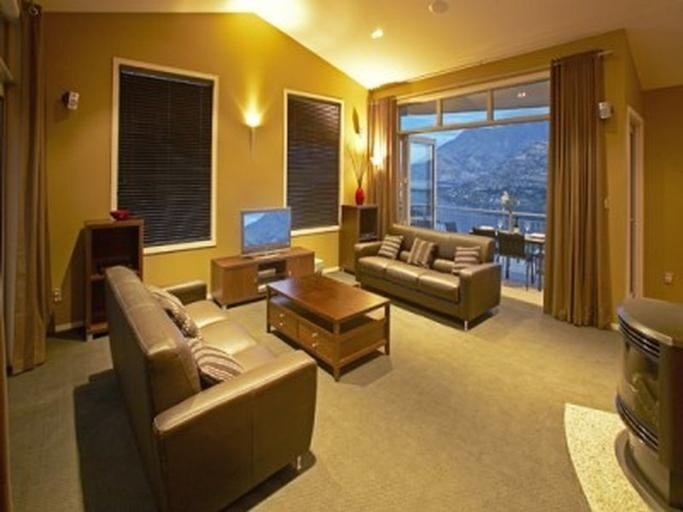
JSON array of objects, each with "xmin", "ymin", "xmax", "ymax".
[
  {"xmin": 149, "ymin": 282, "xmax": 198, "ymax": 338},
  {"xmin": 407, "ymin": 238, "xmax": 439, "ymax": 269},
  {"xmin": 377, "ymin": 233, "xmax": 403, "ymax": 260},
  {"xmin": 187, "ymin": 336, "xmax": 247, "ymax": 383},
  {"xmin": 453, "ymin": 245, "xmax": 482, "ymax": 277}
]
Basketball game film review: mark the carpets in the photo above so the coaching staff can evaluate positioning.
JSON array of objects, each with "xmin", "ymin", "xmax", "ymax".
[{"xmin": 0, "ymin": 267, "xmax": 627, "ymax": 511}]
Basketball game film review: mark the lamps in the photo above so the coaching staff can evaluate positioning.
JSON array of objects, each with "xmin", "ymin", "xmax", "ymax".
[{"xmin": 428, "ymin": 0, "xmax": 448, "ymax": 15}]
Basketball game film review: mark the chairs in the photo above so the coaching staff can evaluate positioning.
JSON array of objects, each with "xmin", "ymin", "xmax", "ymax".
[
  {"xmin": 493, "ymin": 232, "xmax": 535, "ymax": 291},
  {"xmin": 472, "ymin": 228, "xmax": 504, "ymax": 275}
]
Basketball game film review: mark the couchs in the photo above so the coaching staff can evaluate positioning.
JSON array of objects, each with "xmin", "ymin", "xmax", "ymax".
[
  {"xmin": 104, "ymin": 266, "xmax": 318, "ymax": 512},
  {"xmin": 354, "ymin": 222, "xmax": 501, "ymax": 331}
]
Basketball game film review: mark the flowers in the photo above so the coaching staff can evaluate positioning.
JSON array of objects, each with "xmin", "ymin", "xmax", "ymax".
[
  {"xmin": 346, "ymin": 130, "xmax": 373, "ymax": 183},
  {"xmin": 499, "ymin": 190, "xmax": 518, "ymax": 213}
]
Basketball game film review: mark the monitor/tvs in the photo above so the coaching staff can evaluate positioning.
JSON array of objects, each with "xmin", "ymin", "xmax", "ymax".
[{"xmin": 238, "ymin": 206, "xmax": 293, "ymax": 260}]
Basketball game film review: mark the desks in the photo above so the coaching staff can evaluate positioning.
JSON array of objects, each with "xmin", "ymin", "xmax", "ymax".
[{"xmin": 470, "ymin": 228, "xmax": 544, "ymax": 292}]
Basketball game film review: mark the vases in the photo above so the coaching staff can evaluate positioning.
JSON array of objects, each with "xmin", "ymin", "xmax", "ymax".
[
  {"xmin": 508, "ymin": 214, "xmax": 513, "ymax": 231},
  {"xmin": 354, "ymin": 182, "xmax": 366, "ymax": 202}
]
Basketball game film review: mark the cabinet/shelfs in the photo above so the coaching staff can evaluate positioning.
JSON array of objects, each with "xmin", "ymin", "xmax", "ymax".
[
  {"xmin": 338, "ymin": 204, "xmax": 379, "ymax": 273},
  {"xmin": 83, "ymin": 217, "xmax": 146, "ymax": 338},
  {"xmin": 210, "ymin": 245, "xmax": 315, "ymax": 312}
]
